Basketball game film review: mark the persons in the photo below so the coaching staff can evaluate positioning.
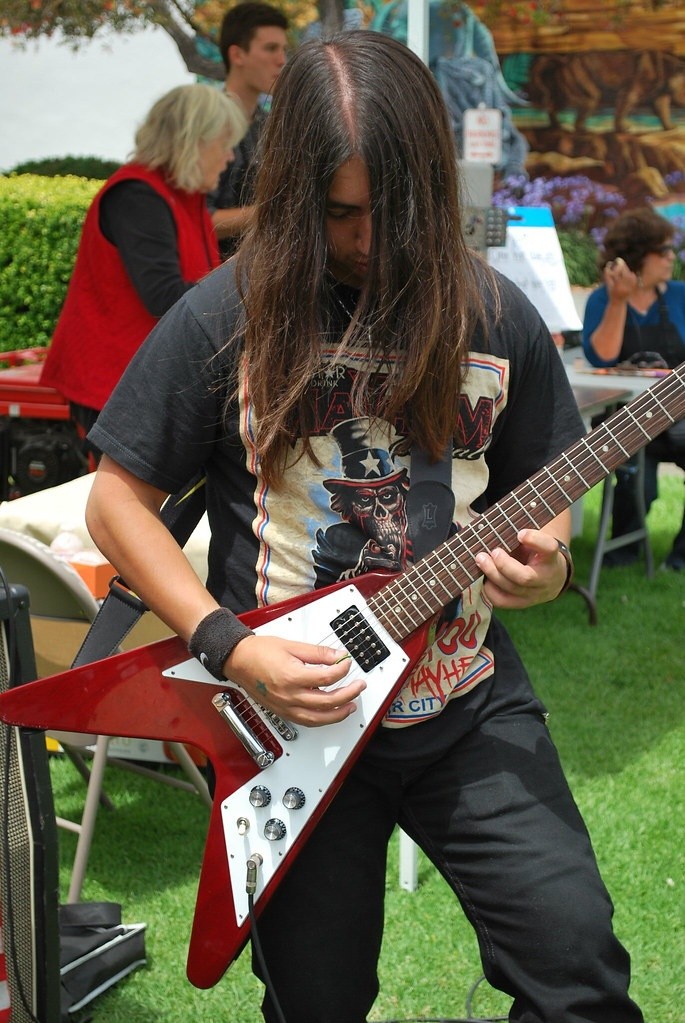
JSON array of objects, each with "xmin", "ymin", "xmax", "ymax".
[
  {"xmin": 39, "ymin": 86, "xmax": 247, "ymax": 470},
  {"xmin": 581, "ymin": 206, "xmax": 685, "ymax": 571},
  {"xmin": 84, "ymin": 30, "xmax": 641, "ymax": 1023},
  {"xmin": 207, "ymin": 2, "xmax": 290, "ymax": 262}
]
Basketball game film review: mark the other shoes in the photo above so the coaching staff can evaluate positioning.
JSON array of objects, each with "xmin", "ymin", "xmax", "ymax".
[{"xmin": 603, "ymin": 550, "xmax": 639, "ymax": 567}]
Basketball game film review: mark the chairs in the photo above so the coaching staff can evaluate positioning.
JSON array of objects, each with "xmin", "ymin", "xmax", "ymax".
[{"xmin": 0, "ymin": 532, "xmax": 214, "ymax": 903}]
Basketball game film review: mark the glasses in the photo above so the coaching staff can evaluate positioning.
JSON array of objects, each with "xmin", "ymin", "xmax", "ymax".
[{"xmin": 647, "ymin": 245, "xmax": 676, "ymax": 258}]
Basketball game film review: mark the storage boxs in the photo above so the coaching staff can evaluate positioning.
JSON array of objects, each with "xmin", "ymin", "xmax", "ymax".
[{"xmin": 70, "ymin": 563, "xmax": 123, "ymax": 599}]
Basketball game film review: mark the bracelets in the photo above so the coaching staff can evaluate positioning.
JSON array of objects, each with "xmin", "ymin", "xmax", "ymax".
[
  {"xmin": 187, "ymin": 608, "xmax": 257, "ymax": 683},
  {"xmin": 547, "ymin": 538, "xmax": 573, "ymax": 603}
]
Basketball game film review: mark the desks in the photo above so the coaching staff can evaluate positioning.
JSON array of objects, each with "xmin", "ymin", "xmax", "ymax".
[{"xmin": 560, "ymin": 346, "xmax": 661, "ymax": 599}]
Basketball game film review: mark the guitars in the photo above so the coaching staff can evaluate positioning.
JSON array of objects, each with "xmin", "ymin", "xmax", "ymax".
[{"xmin": 0, "ymin": 360, "xmax": 685, "ymax": 991}]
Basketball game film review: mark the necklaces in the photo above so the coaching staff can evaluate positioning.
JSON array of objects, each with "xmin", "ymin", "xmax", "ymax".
[{"xmin": 332, "ymin": 292, "xmax": 376, "ymax": 362}]
